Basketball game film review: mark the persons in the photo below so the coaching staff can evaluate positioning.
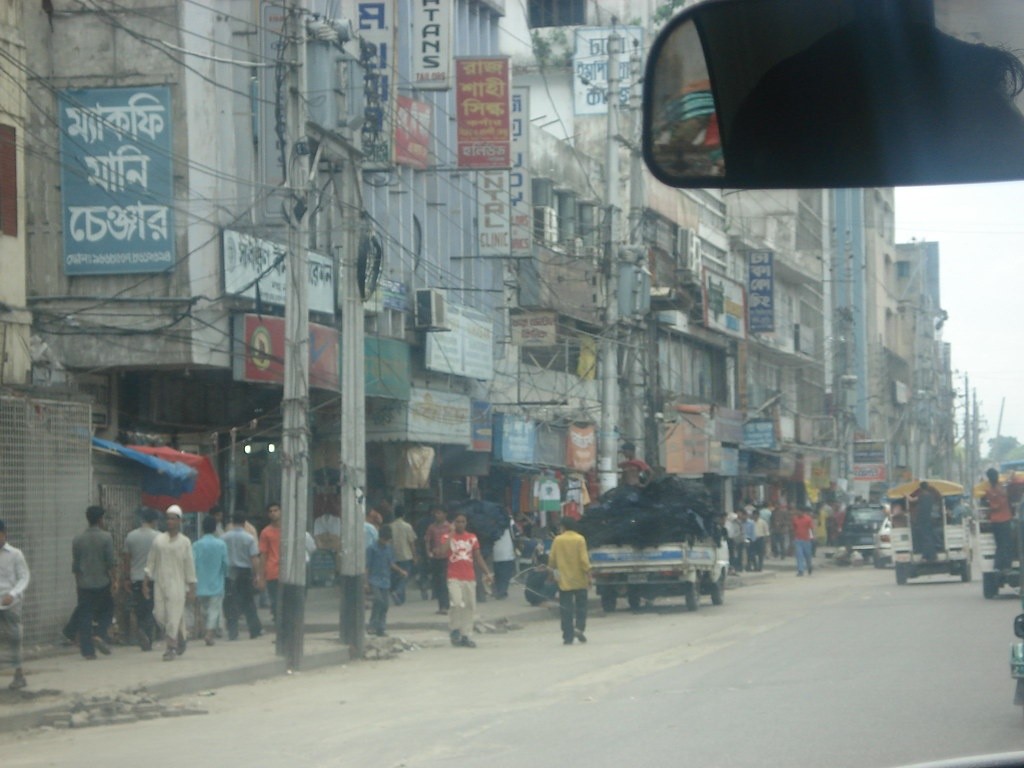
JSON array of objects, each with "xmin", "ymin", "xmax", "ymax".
[
  {"xmin": 981, "ymin": 466, "xmax": 1017, "ymax": 571},
  {"xmin": 720, "ymin": 499, "xmax": 847, "ymax": 577},
  {"xmin": 0, "ymin": 519, "xmax": 29, "ymax": 689},
  {"xmin": 125, "ymin": 502, "xmax": 281, "ymax": 660},
  {"xmin": 364, "ymin": 502, "xmax": 491, "ymax": 648},
  {"xmin": 890, "ymin": 481, "xmax": 971, "ymax": 566},
  {"xmin": 617, "ymin": 442, "xmax": 655, "ymax": 491},
  {"xmin": 494, "ymin": 513, "xmax": 558, "ymax": 610},
  {"xmin": 60, "ymin": 505, "xmax": 117, "ymax": 660},
  {"xmin": 547, "ymin": 516, "xmax": 594, "ymax": 645}
]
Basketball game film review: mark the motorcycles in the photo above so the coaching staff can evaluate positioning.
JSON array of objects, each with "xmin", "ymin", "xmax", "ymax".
[
  {"xmin": 888, "ymin": 479, "xmax": 975, "ymax": 585},
  {"xmin": 975, "ymin": 461, "xmax": 1024, "ymax": 600}
]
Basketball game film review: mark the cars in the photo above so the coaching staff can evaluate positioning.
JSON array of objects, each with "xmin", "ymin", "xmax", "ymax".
[{"xmin": 842, "ymin": 503, "xmax": 892, "ymax": 568}]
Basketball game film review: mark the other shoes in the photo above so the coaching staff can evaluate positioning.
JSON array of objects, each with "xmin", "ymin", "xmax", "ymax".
[
  {"xmin": 92, "ymin": 636, "xmax": 111, "ymax": 655},
  {"xmin": 9, "ymin": 678, "xmax": 26, "ymax": 689},
  {"xmin": 177, "ymin": 637, "xmax": 185, "ymax": 656},
  {"xmin": 448, "ymin": 629, "xmax": 476, "ymax": 647},
  {"xmin": 163, "ymin": 651, "xmax": 174, "ymax": 663},
  {"xmin": 137, "ymin": 630, "xmax": 152, "ymax": 651},
  {"xmin": 573, "ymin": 628, "xmax": 586, "ymax": 643},
  {"xmin": 368, "ymin": 629, "xmax": 389, "ymax": 637}
]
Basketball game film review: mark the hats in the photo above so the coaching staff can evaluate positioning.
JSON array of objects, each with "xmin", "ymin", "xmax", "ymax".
[{"xmin": 166, "ymin": 505, "xmax": 182, "ymax": 519}]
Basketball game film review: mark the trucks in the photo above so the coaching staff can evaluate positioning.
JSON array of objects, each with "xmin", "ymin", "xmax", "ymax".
[{"xmin": 587, "ymin": 524, "xmax": 730, "ymax": 613}]
